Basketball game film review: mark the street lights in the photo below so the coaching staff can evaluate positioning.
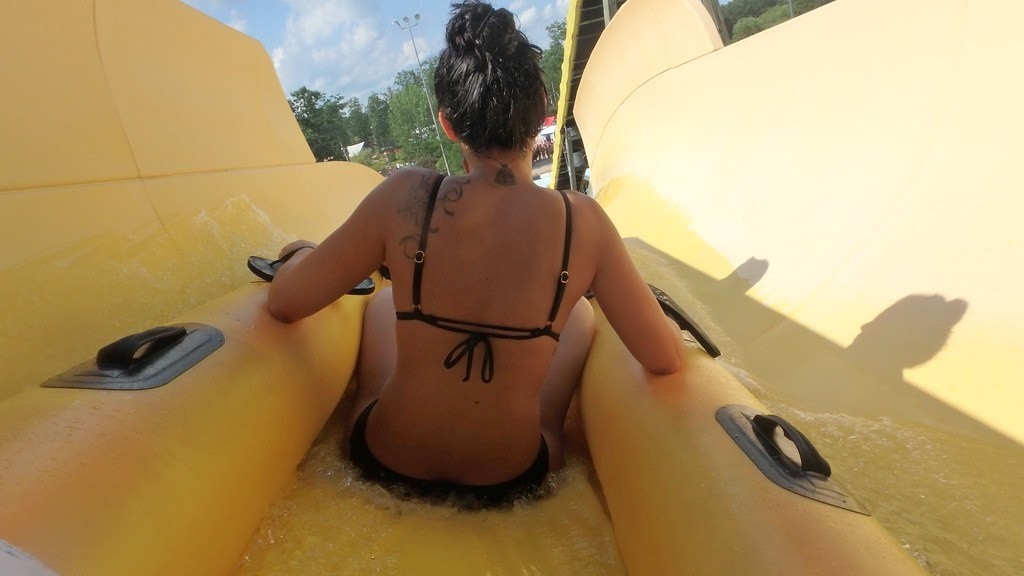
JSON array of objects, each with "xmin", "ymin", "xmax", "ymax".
[{"xmin": 392, "ymin": 13, "xmax": 452, "ymax": 177}]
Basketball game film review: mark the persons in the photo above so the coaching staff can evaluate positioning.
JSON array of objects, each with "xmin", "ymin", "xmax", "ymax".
[{"xmin": 267, "ymin": 0, "xmax": 682, "ymax": 510}]
